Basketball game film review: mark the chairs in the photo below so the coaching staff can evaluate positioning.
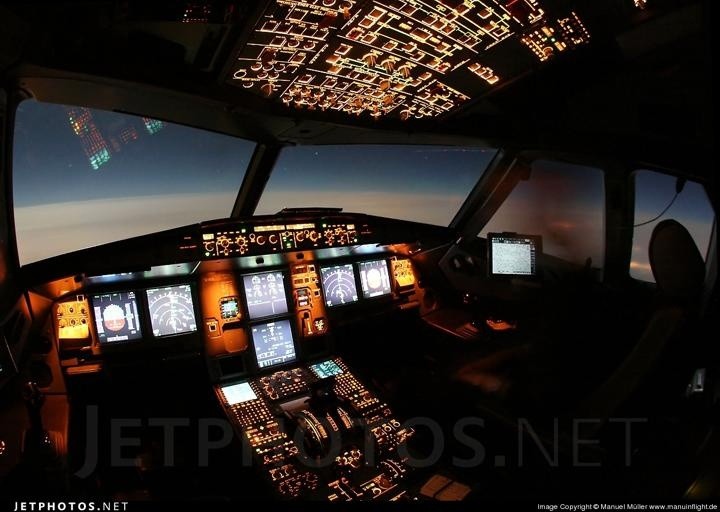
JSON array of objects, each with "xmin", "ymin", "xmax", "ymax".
[{"xmin": 556, "ymin": 215, "xmax": 707, "ymax": 451}]
